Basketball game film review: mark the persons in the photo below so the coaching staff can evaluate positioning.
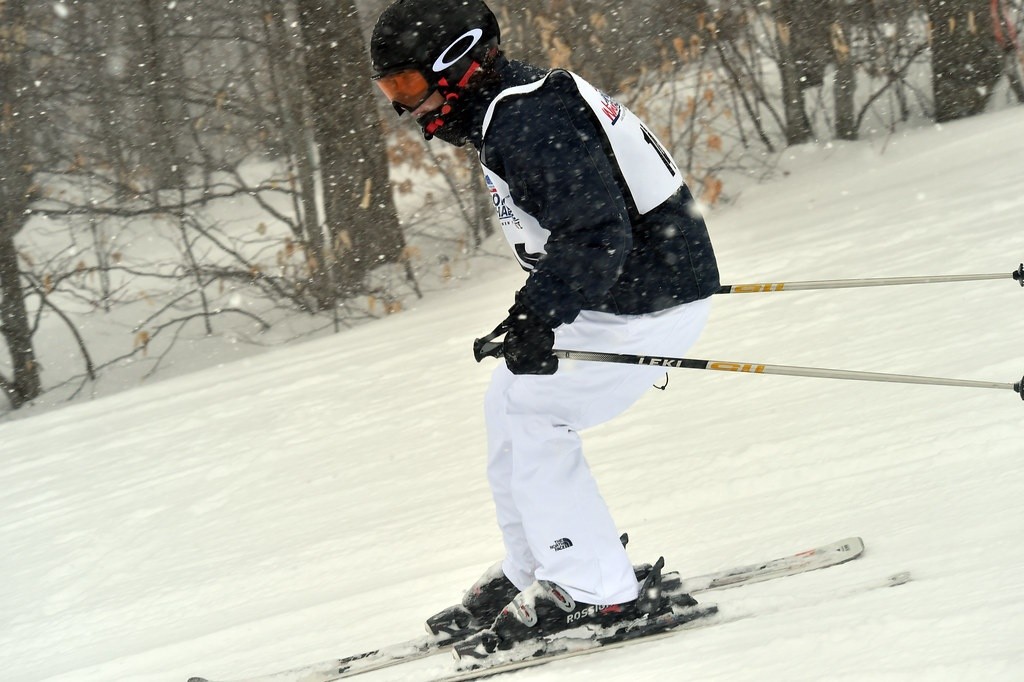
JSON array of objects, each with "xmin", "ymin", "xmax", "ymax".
[{"xmin": 370, "ymin": 0, "xmax": 717, "ymax": 650}]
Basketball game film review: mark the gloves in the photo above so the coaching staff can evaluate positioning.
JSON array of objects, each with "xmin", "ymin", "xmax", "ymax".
[{"xmin": 503, "ymin": 300, "xmax": 559, "ymax": 376}]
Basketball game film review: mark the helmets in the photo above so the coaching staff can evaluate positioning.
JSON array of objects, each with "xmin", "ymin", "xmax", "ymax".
[{"xmin": 368, "ymin": 0, "xmax": 501, "ymax": 147}]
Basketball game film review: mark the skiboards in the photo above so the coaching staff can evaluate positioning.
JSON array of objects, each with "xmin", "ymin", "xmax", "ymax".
[{"xmin": 187, "ymin": 537, "xmax": 946, "ymax": 682}]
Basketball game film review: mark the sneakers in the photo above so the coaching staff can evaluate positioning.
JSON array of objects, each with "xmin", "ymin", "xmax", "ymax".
[
  {"xmin": 462, "ymin": 559, "xmax": 523, "ymax": 626},
  {"xmin": 490, "ymin": 578, "xmax": 643, "ymax": 651}
]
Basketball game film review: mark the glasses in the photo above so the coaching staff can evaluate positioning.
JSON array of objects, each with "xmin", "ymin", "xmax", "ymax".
[{"xmin": 371, "ymin": 67, "xmax": 434, "ymax": 117}]
